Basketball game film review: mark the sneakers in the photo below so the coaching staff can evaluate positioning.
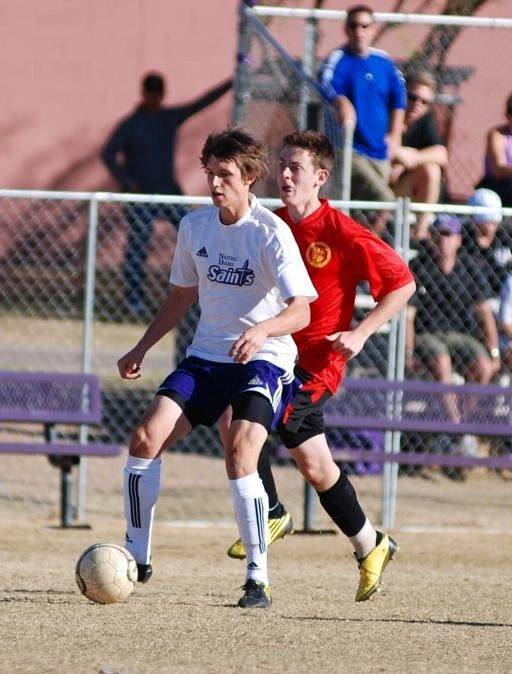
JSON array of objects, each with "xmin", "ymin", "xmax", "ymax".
[
  {"xmin": 237, "ymin": 578, "xmax": 272, "ymax": 608},
  {"xmin": 458, "ymin": 434, "xmax": 477, "ymax": 480},
  {"xmin": 350, "ymin": 530, "xmax": 399, "ymax": 603},
  {"xmin": 136, "ymin": 562, "xmax": 152, "ymax": 584},
  {"xmin": 227, "ymin": 502, "xmax": 294, "ymax": 560}
]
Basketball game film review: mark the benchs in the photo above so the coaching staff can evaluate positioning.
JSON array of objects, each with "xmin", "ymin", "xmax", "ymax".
[
  {"xmin": 347, "ymin": 195, "xmax": 510, "ymax": 375},
  {"xmin": 264, "ymin": 375, "xmax": 511, "ymax": 533},
  {"xmin": 1, "ymin": 372, "xmax": 123, "ymax": 527}
]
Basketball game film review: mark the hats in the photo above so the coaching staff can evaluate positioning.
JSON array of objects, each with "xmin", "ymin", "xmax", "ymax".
[
  {"xmin": 466, "ymin": 187, "xmax": 503, "ymax": 221},
  {"xmin": 430, "ymin": 213, "xmax": 461, "ymax": 234}
]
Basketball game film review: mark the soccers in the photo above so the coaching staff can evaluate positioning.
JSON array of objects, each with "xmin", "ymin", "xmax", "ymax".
[{"xmin": 75, "ymin": 543, "xmax": 140, "ymax": 604}]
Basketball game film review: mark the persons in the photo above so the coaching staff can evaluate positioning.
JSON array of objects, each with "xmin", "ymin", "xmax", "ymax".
[
  {"xmin": 225, "ymin": 128, "xmax": 419, "ymax": 605},
  {"xmin": 498, "ymin": 273, "xmax": 511, "ymax": 372},
  {"xmin": 473, "ymin": 88, "xmax": 512, "ymax": 206},
  {"xmin": 389, "ymin": 68, "xmax": 448, "ymax": 240},
  {"xmin": 459, "ymin": 186, "xmax": 511, "ymax": 319},
  {"xmin": 117, "ymin": 122, "xmax": 321, "ymax": 611},
  {"xmin": 403, "ymin": 211, "xmax": 503, "ymax": 422},
  {"xmin": 312, "ymin": 3, "xmax": 409, "ymax": 237},
  {"xmin": 94, "ymin": 67, "xmax": 237, "ymax": 322}
]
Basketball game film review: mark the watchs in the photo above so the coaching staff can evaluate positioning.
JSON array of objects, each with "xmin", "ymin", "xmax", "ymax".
[{"xmin": 488, "ymin": 346, "xmax": 501, "ymax": 360}]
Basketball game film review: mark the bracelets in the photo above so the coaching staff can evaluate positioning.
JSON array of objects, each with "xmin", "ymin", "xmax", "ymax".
[{"xmin": 404, "ymin": 348, "xmax": 415, "ymax": 357}]
